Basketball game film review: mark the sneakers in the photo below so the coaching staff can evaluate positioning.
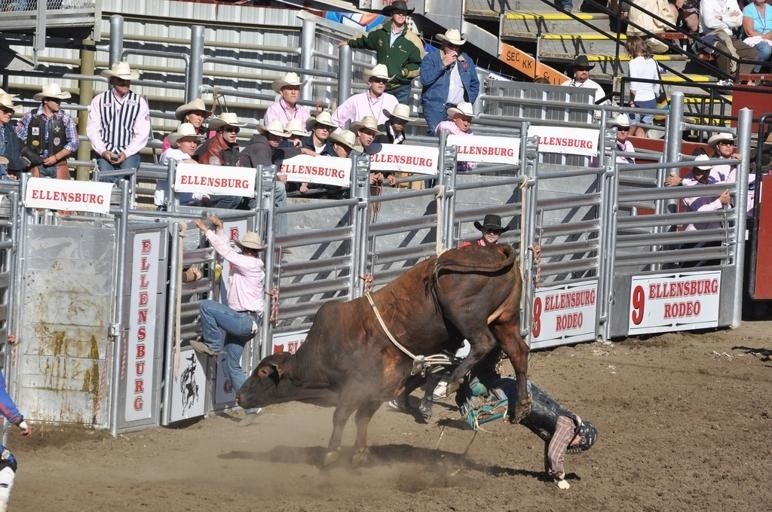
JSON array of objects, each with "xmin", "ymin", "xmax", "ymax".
[
  {"xmin": 190, "ymin": 340, "xmax": 218, "ymax": 356},
  {"xmin": 224, "ymin": 405, "xmax": 245, "ymax": 418},
  {"xmin": 389, "ymin": 400, "xmax": 409, "ymax": 412},
  {"xmin": 432, "ymin": 381, "xmax": 448, "ymax": 399}
]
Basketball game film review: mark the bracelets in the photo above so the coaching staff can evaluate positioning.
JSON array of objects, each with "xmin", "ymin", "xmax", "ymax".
[{"xmin": 192, "ymin": 271, "xmax": 199, "ymax": 281}]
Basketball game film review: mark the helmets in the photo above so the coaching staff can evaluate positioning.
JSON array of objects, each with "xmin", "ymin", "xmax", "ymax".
[{"xmin": 579, "ymin": 421, "xmax": 597, "ymax": 451}]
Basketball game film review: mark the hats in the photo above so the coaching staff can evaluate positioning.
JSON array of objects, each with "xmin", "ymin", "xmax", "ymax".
[
  {"xmin": 474, "ymin": 215, "xmax": 509, "ymax": 232},
  {"xmin": 362, "ymin": 64, "xmax": 397, "ymax": 82},
  {"xmin": 607, "ymin": 114, "xmax": 630, "ymax": 126},
  {"xmin": 435, "ymin": 28, "xmax": 467, "ymax": 46},
  {"xmin": 257, "ymin": 104, "xmax": 416, "ymax": 153},
  {"xmin": 694, "ymin": 154, "xmax": 713, "ymax": 170},
  {"xmin": 209, "ymin": 112, "xmax": 247, "ymax": 128},
  {"xmin": 33, "ymin": 82, "xmax": 72, "ymax": 101},
  {"xmin": 383, "ymin": 1, "xmax": 415, "ymax": 16},
  {"xmin": 101, "ymin": 62, "xmax": 139, "ymax": 80},
  {"xmin": 708, "ymin": 132, "xmax": 738, "ymax": 144},
  {"xmin": 566, "ymin": 55, "xmax": 595, "ymax": 70},
  {"xmin": 447, "ymin": 103, "xmax": 476, "ymax": 117},
  {"xmin": 272, "ymin": 72, "xmax": 308, "ymax": 93},
  {"xmin": 168, "ymin": 99, "xmax": 212, "ymax": 145},
  {"xmin": 0, "ymin": 93, "xmax": 23, "ymax": 111},
  {"xmin": 235, "ymin": 231, "xmax": 267, "ymax": 251}
]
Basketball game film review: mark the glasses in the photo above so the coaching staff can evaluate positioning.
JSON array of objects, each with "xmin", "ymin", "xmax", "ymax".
[
  {"xmin": 374, "ymin": 78, "xmax": 388, "ymax": 83},
  {"xmin": 618, "ymin": 127, "xmax": 630, "ymax": 131},
  {"xmin": 227, "ymin": 127, "xmax": 240, "ymax": 132}
]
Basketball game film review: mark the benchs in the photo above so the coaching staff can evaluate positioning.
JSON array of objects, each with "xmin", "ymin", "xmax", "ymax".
[{"xmin": 589, "ymin": 0, "xmax": 772, "ymax": 88}]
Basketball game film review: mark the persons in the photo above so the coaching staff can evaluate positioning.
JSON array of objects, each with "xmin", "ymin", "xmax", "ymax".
[
  {"xmin": 1, "ymin": 368, "xmax": 31, "ymax": 511},
  {"xmin": 85, "ymin": 62, "xmax": 151, "ymax": 183},
  {"xmin": 626, "ymin": 33, "xmax": 661, "ymax": 137},
  {"xmin": 155, "ymin": 72, "xmax": 410, "ymax": 209},
  {"xmin": 464, "ymin": 376, "xmax": 598, "ymax": 489},
  {"xmin": 180, "ymin": 266, "xmax": 205, "ymax": 284},
  {"xmin": 191, "ymin": 213, "xmax": 268, "ymax": 420},
  {"xmin": 666, "ymin": 130, "xmax": 763, "ymax": 247},
  {"xmin": 435, "ymin": 102, "xmax": 479, "ymax": 172},
  {"xmin": 2, "ymin": 81, "xmax": 80, "ymax": 179},
  {"xmin": 431, "ymin": 215, "xmax": 511, "ymax": 399},
  {"xmin": 419, "ymin": 29, "xmax": 480, "ymax": 135},
  {"xmin": 338, "ymin": 2, "xmax": 425, "ymax": 104},
  {"xmin": 583, "ymin": 0, "xmax": 772, "ymax": 86},
  {"xmin": 560, "ymin": 54, "xmax": 636, "ymax": 165},
  {"xmin": 330, "ymin": 64, "xmax": 398, "ymax": 130}
]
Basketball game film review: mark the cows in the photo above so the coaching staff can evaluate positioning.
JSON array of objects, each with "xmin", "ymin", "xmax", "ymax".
[{"xmin": 234, "ymin": 241, "xmax": 535, "ymax": 468}]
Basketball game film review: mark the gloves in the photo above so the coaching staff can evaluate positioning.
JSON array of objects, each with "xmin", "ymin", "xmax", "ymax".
[{"xmin": 558, "ymin": 479, "xmax": 570, "ymax": 489}]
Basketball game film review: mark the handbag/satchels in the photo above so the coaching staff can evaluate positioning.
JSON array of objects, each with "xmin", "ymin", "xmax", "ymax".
[{"xmin": 654, "ymin": 92, "xmax": 669, "ymax": 121}]
